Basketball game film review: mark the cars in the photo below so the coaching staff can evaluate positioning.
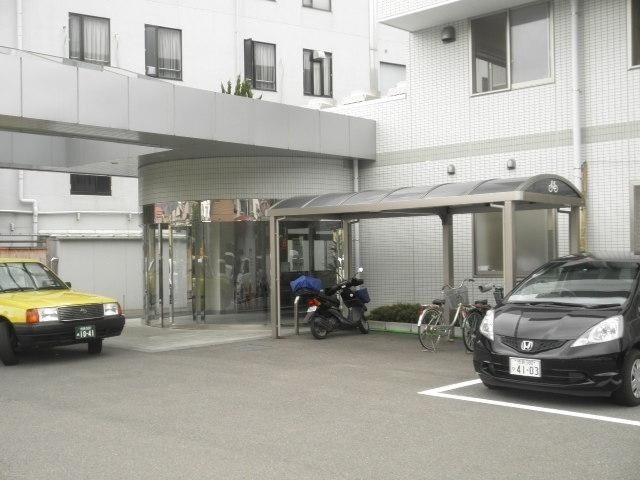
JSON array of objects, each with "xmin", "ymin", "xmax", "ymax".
[
  {"xmin": 473, "ymin": 251, "xmax": 640, "ymax": 406},
  {"xmin": 0, "ymin": 258, "xmax": 125, "ymax": 365}
]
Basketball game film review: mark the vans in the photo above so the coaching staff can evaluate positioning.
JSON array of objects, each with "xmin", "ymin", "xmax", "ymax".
[{"xmin": 237, "ymin": 250, "xmax": 300, "ymax": 303}]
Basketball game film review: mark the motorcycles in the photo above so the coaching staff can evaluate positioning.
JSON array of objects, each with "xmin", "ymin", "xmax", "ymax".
[{"xmin": 289, "ymin": 267, "xmax": 369, "ymax": 339}]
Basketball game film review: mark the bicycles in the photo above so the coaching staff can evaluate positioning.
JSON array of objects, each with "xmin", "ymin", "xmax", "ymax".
[{"xmin": 418, "ymin": 278, "xmax": 504, "ymax": 354}]
